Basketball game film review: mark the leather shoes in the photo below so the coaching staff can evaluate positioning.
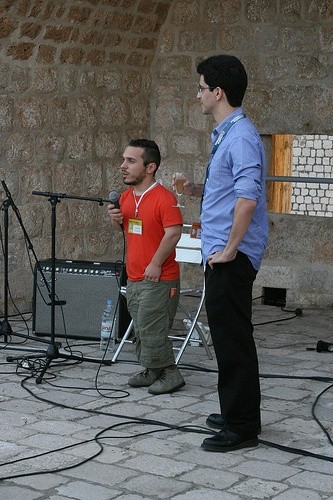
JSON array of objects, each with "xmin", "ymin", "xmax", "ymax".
[
  {"xmin": 201, "ymin": 428, "xmax": 259, "ymax": 452},
  {"xmin": 205, "ymin": 414, "xmax": 225, "ymax": 429}
]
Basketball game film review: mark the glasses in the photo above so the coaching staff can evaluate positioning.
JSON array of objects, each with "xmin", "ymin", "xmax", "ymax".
[{"xmin": 198, "ymin": 85, "xmax": 224, "ymax": 93}]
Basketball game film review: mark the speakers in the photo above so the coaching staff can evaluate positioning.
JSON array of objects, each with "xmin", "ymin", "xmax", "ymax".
[{"xmin": 32, "ymin": 258, "xmax": 133, "ymax": 341}]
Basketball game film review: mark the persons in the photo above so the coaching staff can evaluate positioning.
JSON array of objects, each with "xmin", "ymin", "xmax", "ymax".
[
  {"xmin": 172, "ymin": 54, "xmax": 269, "ymax": 452},
  {"xmin": 107, "ymin": 139, "xmax": 186, "ymax": 394}
]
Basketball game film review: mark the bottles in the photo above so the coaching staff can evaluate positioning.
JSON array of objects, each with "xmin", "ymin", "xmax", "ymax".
[
  {"xmin": 191, "ymin": 221, "xmax": 201, "ymax": 238},
  {"xmin": 100, "ymin": 300, "xmax": 115, "ymax": 352}
]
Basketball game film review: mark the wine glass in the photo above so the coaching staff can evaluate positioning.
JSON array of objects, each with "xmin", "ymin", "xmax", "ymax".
[{"xmin": 172, "ymin": 171, "xmax": 186, "ymax": 209}]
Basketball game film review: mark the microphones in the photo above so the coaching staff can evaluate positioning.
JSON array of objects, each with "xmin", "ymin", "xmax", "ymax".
[
  {"xmin": 108, "ymin": 191, "xmax": 124, "ymax": 229},
  {"xmin": 47, "ymin": 300, "xmax": 66, "ymax": 306}
]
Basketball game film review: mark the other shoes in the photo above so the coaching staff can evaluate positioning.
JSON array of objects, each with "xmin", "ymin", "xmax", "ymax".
[
  {"xmin": 127, "ymin": 368, "xmax": 163, "ymax": 387},
  {"xmin": 148, "ymin": 365, "xmax": 185, "ymax": 395}
]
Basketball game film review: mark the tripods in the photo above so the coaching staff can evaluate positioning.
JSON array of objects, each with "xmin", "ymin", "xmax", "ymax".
[{"xmin": 0, "ymin": 178, "xmax": 111, "ymax": 385}]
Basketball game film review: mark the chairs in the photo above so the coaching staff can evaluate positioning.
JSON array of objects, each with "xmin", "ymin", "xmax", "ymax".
[{"xmin": 112, "ymin": 224, "xmax": 214, "ymax": 365}]
